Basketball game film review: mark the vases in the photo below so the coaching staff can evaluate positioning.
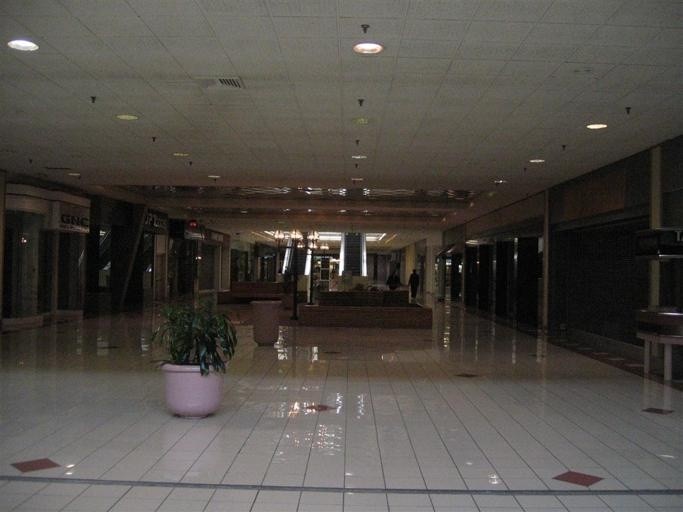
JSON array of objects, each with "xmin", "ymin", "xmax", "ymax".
[{"xmin": 250, "ymin": 299, "xmax": 282, "ymax": 347}]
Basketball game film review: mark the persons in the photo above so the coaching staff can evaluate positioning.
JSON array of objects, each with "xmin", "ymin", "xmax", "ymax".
[
  {"xmin": 408, "ymin": 268, "xmax": 420, "ymax": 299},
  {"xmin": 387, "ymin": 269, "xmax": 400, "ymax": 291}
]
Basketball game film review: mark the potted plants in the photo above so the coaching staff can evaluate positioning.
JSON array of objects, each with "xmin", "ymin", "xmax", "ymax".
[{"xmin": 150, "ymin": 294, "xmax": 237, "ymax": 422}]
